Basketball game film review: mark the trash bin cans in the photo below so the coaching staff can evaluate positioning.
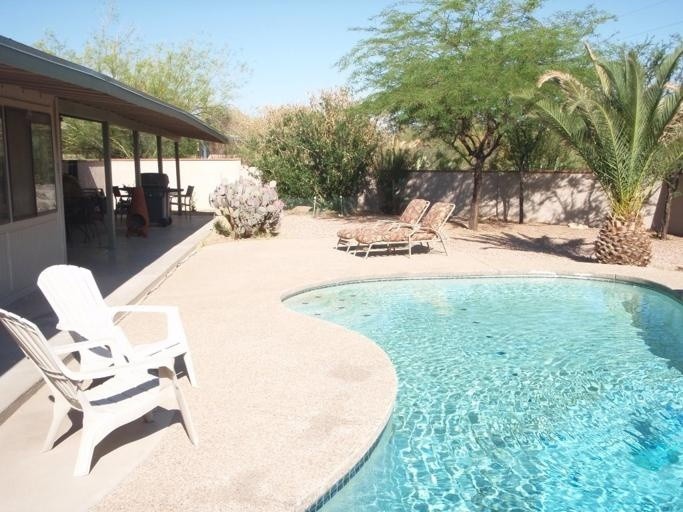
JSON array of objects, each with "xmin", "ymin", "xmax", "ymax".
[{"xmin": 141, "ymin": 173, "xmax": 171, "ymax": 226}]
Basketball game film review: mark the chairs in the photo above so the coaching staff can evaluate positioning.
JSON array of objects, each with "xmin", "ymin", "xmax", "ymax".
[
  {"xmin": 111, "ymin": 184, "xmax": 195, "ymax": 217},
  {"xmin": 62, "ymin": 173, "xmax": 107, "ymax": 242},
  {"xmin": 335, "ymin": 198, "xmax": 456, "ymax": 260},
  {"xmin": 1, "ymin": 309, "xmax": 197, "ymax": 476},
  {"xmin": 37, "ymin": 264, "xmax": 196, "ymax": 390}
]
